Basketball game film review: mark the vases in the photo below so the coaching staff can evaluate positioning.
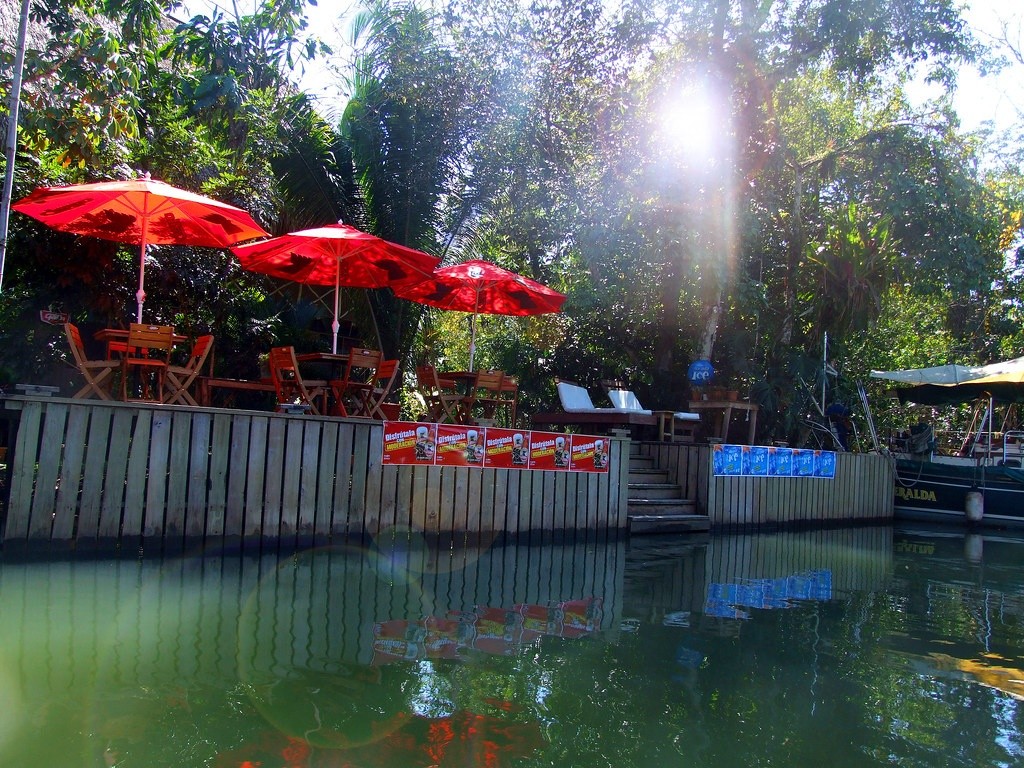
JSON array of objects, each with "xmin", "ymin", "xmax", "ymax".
[{"xmin": 370, "ymin": 402, "xmax": 403, "ymax": 422}]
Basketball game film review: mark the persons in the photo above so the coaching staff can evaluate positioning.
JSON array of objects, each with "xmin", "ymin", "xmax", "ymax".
[{"xmin": 827, "ymin": 397, "xmax": 850, "ymax": 451}]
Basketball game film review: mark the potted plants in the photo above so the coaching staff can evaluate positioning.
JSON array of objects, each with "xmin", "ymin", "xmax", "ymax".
[{"xmin": 688, "ymin": 382, "xmax": 740, "ymax": 401}]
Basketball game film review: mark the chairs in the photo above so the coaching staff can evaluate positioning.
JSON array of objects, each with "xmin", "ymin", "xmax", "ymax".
[
  {"xmin": 64, "ymin": 322, "xmax": 517, "ymax": 428},
  {"xmin": 606, "ymin": 390, "xmax": 704, "ymax": 443},
  {"xmin": 533, "ymin": 382, "xmax": 658, "ymax": 440}
]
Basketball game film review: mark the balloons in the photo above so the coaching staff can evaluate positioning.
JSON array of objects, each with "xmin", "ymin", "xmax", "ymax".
[{"xmin": 688, "ymin": 361, "xmax": 714, "ymax": 385}]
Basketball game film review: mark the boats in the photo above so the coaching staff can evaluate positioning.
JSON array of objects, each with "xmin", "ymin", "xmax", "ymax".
[{"xmin": 869, "ymin": 355, "xmax": 1024, "ymax": 528}]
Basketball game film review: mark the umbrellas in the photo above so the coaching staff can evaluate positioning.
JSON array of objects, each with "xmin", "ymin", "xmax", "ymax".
[
  {"xmin": 11, "ymin": 170, "xmax": 273, "ymax": 400},
  {"xmin": 229, "ymin": 219, "xmax": 443, "ymax": 356},
  {"xmin": 394, "ymin": 253, "xmax": 569, "ymax": 370}
]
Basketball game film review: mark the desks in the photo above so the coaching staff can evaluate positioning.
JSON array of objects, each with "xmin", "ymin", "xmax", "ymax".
[
  {"xmin": 438, "ymin": 371, "xmax": 480, "ymax": 426},
  {"xmin": 93, "ymin": 328, "xmax": 188, "ymax": 400},
  {"xmin": 651, "ymin": 410, "xmax": 677, "ymax": 443},
  {"xmin": 296, "ymin": 353, "xmax": 349, "ymax": 416},
  {"xmin": 686, "ymin": 399, "xmax": 760, "ymax": 445}
]
